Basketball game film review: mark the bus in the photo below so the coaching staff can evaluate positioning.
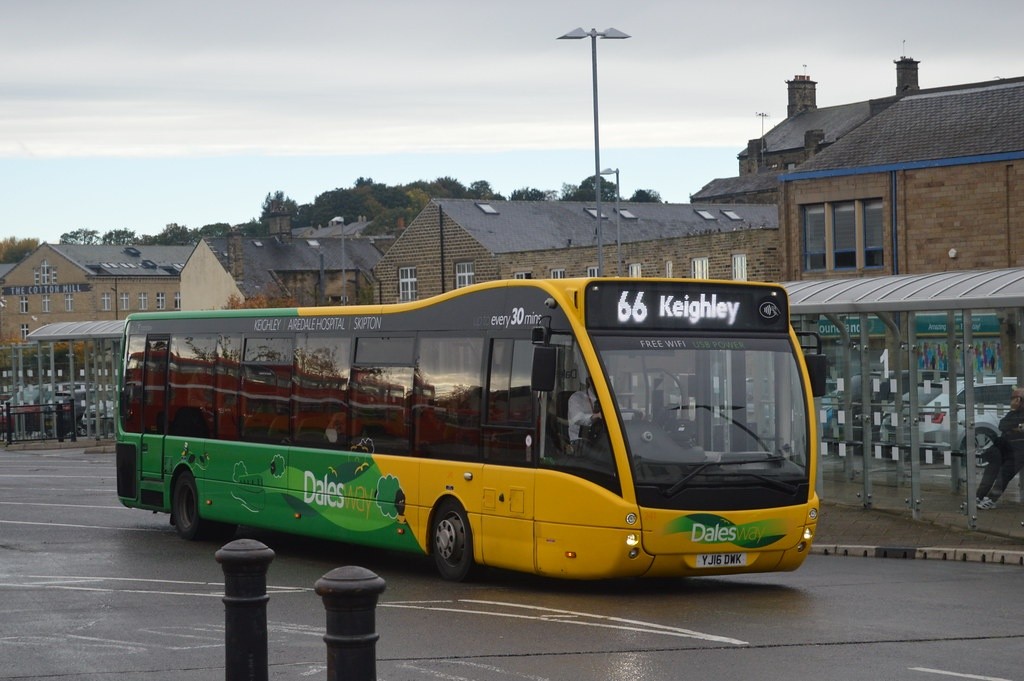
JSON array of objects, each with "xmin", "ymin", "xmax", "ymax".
[
  {"xmin": 125, "ymin": 350, "xmax": 539, "ymax": 451},
  {"xmin": 116, "ymin": 277, "xmax": 826, "ymax": 586}
]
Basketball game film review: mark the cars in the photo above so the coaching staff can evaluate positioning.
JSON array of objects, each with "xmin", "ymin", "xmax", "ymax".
[{"xmin": 0, "ymin": 381, "xmax": 117, "ymax": 437}]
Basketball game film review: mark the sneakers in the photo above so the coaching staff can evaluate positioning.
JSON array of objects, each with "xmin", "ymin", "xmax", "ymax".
[
  {"xmin": 963, "ymin": 498, "xmax": 980, "ymax": 505},
  {"xmin": 977, "ymin": 497, "xmax": 996, "ymax": 509}
]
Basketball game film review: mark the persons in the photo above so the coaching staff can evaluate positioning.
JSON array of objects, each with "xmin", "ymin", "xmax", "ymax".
[
  {"xmin": 960, "ymin": 387, "xmax": 1024, "ymax": 510},
  {"xmin": 567, "ymin": 376, "xmax": 603, "ymax": 455}
]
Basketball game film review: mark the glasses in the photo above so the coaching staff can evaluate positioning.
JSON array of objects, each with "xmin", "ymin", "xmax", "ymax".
[{"xmin": 1010, "ymin": 396, "xmax": 1022, "ymax": 401}]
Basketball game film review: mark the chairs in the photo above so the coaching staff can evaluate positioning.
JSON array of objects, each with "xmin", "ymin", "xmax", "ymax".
[{"xmin": 170, "ymin": 404, "xmax": 541, "ymax": 463}]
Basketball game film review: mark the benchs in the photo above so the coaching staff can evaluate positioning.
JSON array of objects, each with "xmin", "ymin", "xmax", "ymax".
[{"xmin": 821, "ymin": 438, "xmax": 1024, "ymax": 509}]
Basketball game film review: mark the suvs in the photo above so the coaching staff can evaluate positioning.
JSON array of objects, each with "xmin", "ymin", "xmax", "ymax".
[
  {"xmin": 821, "ymin": 370, "xmax": 964, "ymax": 449},
  {"xmin": 880, "ymin": 375, "xmax": 1017, "ymax": 469}
]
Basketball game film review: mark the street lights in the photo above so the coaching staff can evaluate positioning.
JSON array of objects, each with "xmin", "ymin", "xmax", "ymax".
[
  {"xmin": 331, "ymin": 217, "xmax": 346, "ymax": 306},
  {"xmin": 556, "ymin": 27, "xmax": 632, "ymax": 278},
  {"xmin": 599, "ymin": 167, "xmax": 623, "ymax": 278}
]
